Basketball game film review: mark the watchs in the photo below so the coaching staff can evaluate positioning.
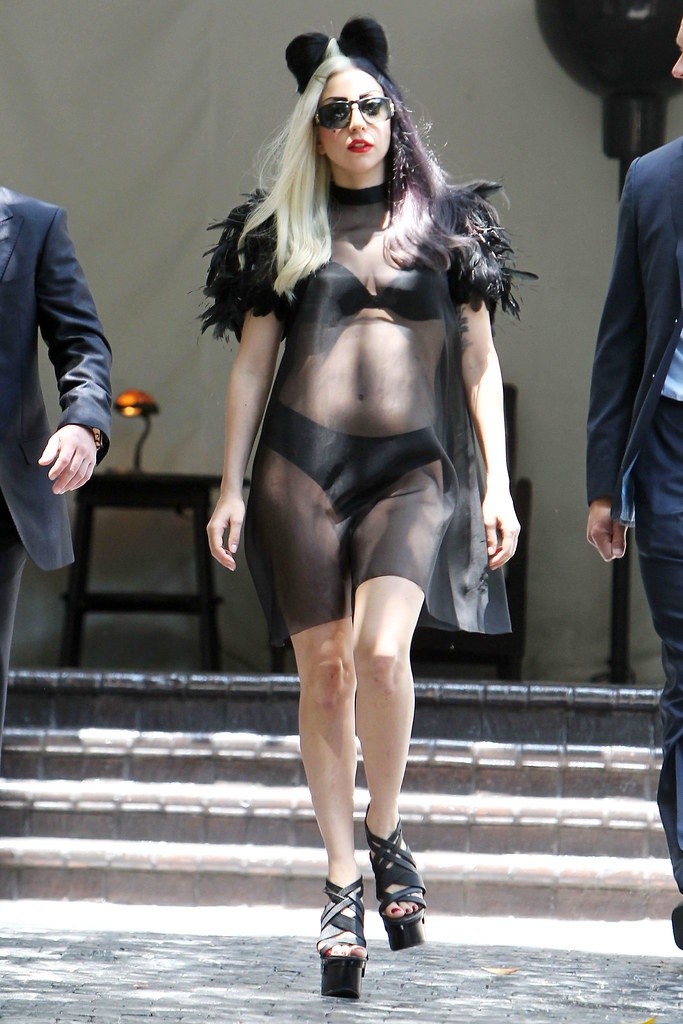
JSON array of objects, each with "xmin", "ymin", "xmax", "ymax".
[{"xmin": 88, "ymin": 425, "xmax": 103, "ymax": 449}]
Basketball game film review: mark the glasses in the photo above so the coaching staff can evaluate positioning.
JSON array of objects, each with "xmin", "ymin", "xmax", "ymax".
[{"xmin": 312, "ymin": 97, "xmax": 396, "ymax": 129}]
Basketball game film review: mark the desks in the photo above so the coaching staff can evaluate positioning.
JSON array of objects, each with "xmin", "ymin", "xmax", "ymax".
[{"xmin": 55, "ymin": 469, "xmax": 252, "ymax": 669}]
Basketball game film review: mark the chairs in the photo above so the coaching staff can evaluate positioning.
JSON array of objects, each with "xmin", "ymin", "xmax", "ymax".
[{"xmin": 409, "ymin": 384, "xmax": 534, "ymax": 681}]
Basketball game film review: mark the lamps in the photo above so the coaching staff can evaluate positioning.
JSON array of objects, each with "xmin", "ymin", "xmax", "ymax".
[{"xmin": 113, "ymin": 387, "xmax": 160, "ymax": 473}]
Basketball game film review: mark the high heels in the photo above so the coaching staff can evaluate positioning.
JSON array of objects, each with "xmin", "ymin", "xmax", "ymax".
[
  {"xmin": 364, "ymin": 803, "xmax": 427, "ymax": 951},
  {"xmin": 317, "ymin": 874, "xmax": 369, "ymax": 999}
]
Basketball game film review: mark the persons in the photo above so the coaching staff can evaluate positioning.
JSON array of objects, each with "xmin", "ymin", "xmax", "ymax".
[
  {"xmin": 0, "ymin": 183, "xmax": 112, "ymax": 748},
  {"xmin": 585, "ymin": 13, "xmax": 683, "ymax": 954},
  {"xmin": 198, "ymin": 18, "xmax": 539, "ymax": 998}
]
